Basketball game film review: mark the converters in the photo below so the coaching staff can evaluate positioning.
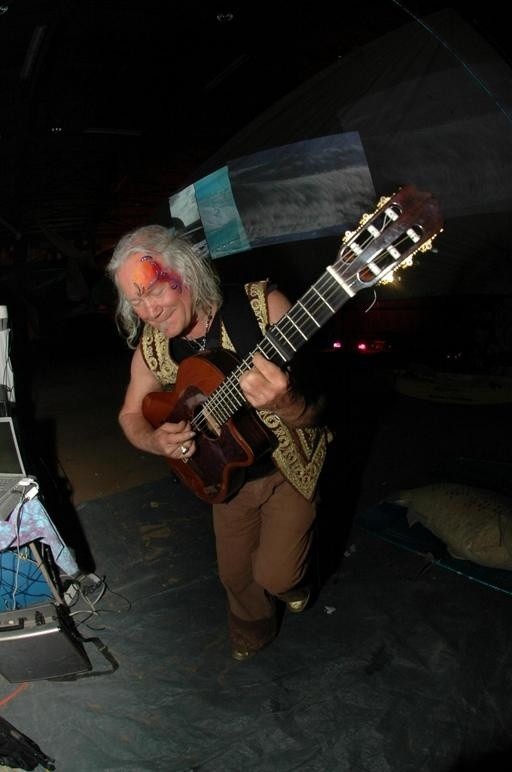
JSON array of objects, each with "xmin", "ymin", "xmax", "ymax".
[{"xmin": 25, "ymin": 487, "xmax": 40, "ymax": 501}]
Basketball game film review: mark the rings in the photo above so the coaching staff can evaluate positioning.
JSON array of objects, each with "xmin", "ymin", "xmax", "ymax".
[{"xmin": 179, "ymin": 445, "xmax": 190, "ymax": 454}]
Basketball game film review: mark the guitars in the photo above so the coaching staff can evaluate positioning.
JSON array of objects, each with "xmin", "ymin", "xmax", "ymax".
[{"xmin": 142, "ymin": 186, "xmax": 445, "ymax": 504}]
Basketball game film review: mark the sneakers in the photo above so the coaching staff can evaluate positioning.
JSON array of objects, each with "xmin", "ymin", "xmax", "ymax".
[
  {"xmin": 231, "ymin": 645, "xmax": 256, "ymax": 661},
  {"xmin": 283, "ymin": 589, "xmax": 310, "ymax": 612}
]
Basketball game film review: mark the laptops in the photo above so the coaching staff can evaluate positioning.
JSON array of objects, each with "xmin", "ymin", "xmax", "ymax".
[{"xmin": 0, "ymin": 416, "xmax": 40, "ymax": 523}]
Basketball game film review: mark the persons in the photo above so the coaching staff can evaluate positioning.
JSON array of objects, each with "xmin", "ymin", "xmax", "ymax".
[{"xmin": 107, "ymin": 225, "xmax": 337, "ymax": 663}]
